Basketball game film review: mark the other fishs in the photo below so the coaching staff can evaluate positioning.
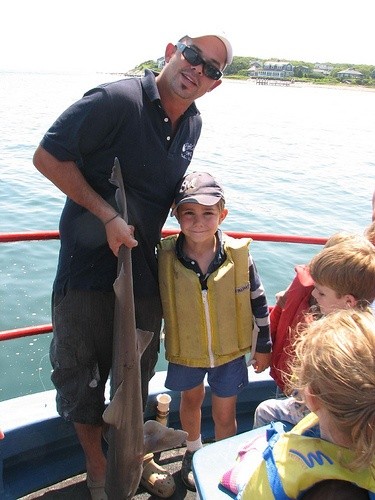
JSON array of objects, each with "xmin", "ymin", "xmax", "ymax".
[{"xmin": 97, "ymin": 155, "xmax": 189, "ymax": 500}]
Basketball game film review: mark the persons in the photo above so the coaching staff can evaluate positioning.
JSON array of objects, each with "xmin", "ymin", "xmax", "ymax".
[
  {"xmin": 31, "ymin": 23, "xmax": 234, "ymax": 500},
  {"xmin": 153, "ymin": 170, "xmax": 274, "ymax": 492},
  {"xmin": 236, "ymin": 303, "xmax": 375, "ymax": 500},
  {"xmin": 248, "ymin": 230, "xmax": 375, "ymax": 429},
  {"xmin": 363, "ymin": 189, "xmax": 375, "ymax": 245}
]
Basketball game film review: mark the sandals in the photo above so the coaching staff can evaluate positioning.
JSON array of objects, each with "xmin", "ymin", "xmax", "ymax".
[
  {"xmin": 86, "ymin": 477, "xmax": 109, "ymax": 500},
  {"xmin": 139, "ymin": 452, "xmax": 175, "ymax": 497}
]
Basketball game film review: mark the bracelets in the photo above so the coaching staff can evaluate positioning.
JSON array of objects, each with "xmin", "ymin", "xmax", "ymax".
[{"xmin": 103, "ymin": 212, "xmax": 121, "ymax": 226}]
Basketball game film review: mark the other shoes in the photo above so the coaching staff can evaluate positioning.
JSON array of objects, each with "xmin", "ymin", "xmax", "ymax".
[{"xmin": 181, "ymin": 449, "xmax": 196, "ymax": 488}]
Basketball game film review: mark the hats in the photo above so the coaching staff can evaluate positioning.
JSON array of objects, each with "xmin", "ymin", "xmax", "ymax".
[
  {"xmin": 170, "ymin": 172, "xmax": 225, "ymax": 217},
  {"xmin": 174, "ymin": 27, "xmax": 233, "ymax": 72}
]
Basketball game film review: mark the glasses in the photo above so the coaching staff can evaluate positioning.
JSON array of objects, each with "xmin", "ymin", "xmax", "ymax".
[{"xmin": 175, "ymin": 42, "xmax": 222, "ymax": 80}]
